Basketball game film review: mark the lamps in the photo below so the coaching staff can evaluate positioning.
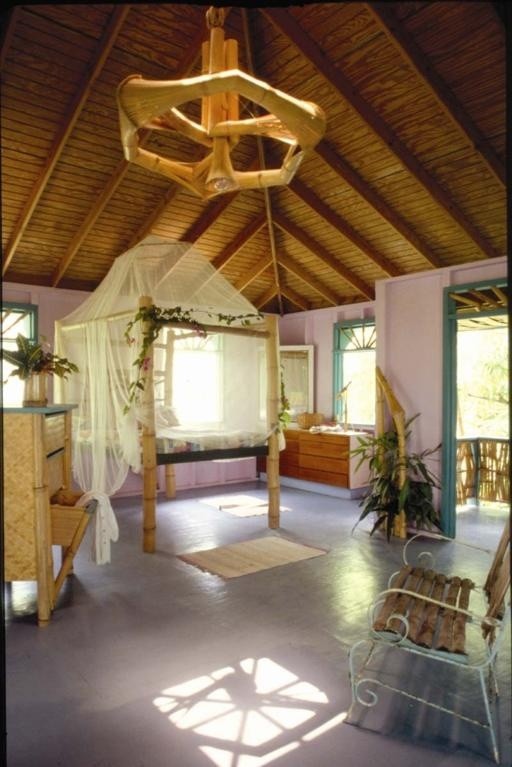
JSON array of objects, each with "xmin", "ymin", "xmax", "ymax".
[{"xmin": 114, "ymin": 0, "xmax": 326, "ymax": 203}]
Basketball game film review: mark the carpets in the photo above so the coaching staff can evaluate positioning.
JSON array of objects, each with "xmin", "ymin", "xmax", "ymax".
[{"xmin": 177, "ymin": 535, "xmax": 329, "ymax": 581}]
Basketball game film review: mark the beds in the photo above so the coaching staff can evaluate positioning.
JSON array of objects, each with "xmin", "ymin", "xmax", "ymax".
[{"xmin": 52, "ymin": 296, "xmax": 281, "ymax": 555}]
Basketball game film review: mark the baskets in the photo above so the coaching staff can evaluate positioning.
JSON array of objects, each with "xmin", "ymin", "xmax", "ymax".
[{"xmin": 297, "ymin": 412, "xmax": 323, "ymax": 429}]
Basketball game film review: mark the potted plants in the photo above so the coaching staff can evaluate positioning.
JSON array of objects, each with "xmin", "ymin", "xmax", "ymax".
[{"xmin": 0, "ymin": 331, "xmax": 81, "ymax": 407}]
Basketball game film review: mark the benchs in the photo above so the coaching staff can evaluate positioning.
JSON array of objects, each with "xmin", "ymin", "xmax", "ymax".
[{"xmin": 343, "ymin": 508, "xmax": 511, "ymax": 767}]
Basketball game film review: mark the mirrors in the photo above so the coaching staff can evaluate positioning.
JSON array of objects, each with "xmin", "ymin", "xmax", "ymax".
[{"xmin": 260, "ymin": 345, "xmax": 315, "ymax": 428}]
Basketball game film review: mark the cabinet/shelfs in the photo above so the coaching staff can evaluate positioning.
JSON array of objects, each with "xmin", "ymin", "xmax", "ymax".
[
  {"xmin": 4, "ymin": 411, "xmax": 99, "ymax": 629},
  {"xmin": 259, "ymin": 429, "xmax": 377, "ymax": 490}
]
